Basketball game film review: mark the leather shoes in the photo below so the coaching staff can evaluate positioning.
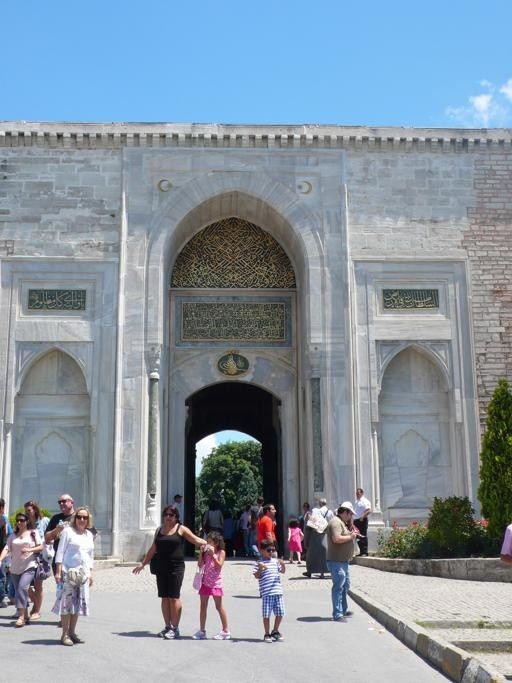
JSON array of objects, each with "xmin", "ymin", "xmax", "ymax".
[
  {"xmin": 356, "ymin": 554, "xmax": 361, "ymax": 556},
  {"xmin": 362, "ymin": 553, "xmax": 367, "ymax": 556}
]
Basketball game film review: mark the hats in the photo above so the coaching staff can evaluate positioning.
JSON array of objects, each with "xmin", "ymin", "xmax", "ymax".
[
  {"xmin": 257, "ymin": 497, "xmax": 264, "ymax": 504},
  {"xmin": 341, "ymin": 501, "xmax": 357, "ymax": 515},
  {"xmin": 261, "ymin": 539, "xmax": 272, "ymax": 549},
  {"xmin": 174, "ymin": 495, "xmax": 183, "ymax": 498}
]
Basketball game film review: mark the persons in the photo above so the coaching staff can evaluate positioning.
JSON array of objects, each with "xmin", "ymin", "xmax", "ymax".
[
  {"xmin": 326, "ymin": 501, "xmax": 360, "ymax": 623},
  {"xmin": 288, "ymin": 517, "xmax": 305, "ymax": 566},
  {"xmin": 301, "ymin": 510, "xmax": 327, "ymax": 579},
  {"xmin": 248, "ymin": 496, "xmax": 265, "ymax": 558},
  {"xmin": 203, "ymin": 501, "xmax": 224, "ymax": 530},
  {"xmin": 256, "ymin": 505, "xmax": 278, "ymax": 558},
  {"xmin": 45, "ymin": 494, "xmax": 99, "ymax": 579},
  {"xmin": 132, "ymin": 504, "xmax": 207, "ymax": 641},
  {"xmin": 240, "ymin": 504, "xmax": 251, "ymax": 556},
  {"xmin": 318, "ymin": 497, "xmax": 333, "ymax": 518},
  {"xmin": 52, "ymin": 508, "xmax": 93, "ymax": 647},
  {"xmin": 191, "ymin": 532, "xmax": 231, "ymax": 642},
  {"xmin": 355, "ymin": 486, "xmax": 372, "ymax": 558},
  {"xmin": 0, "ymin": 513, "xmax": 44, "ymax": 628},
  {"xmin": 500, "ymin": 519, "xmax": 512, "ymax": 567},
  {"xmin": 301, "ymin": 501, "xmax": 312, "ymax": 527},
  {"xmin": 254, "ymin": 538, "xmax": 287, "ymax": 645},
  {"xmin": 222, "ymin": 512, "xmax": 234, "ymax": 557},
  {"xmin": 0, "ymin": 495, "xmax": 10, "ymax": 551},
  {"xmin": 24, "ymin": 500, "xmax": 52, "ymax": 621},
  {"xmin": 173, "ymin": 495, "xmax": 185, "ymax": 525}
]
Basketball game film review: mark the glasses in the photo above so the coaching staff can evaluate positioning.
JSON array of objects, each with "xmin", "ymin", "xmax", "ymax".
[
  {"xmin": 25, "ymin": 501, "xmax": 32, "ymax": 505},
  {"xmin": 58, "ymin": 499, "xmax": 71, "ymax": 503},
  {"xmin": 267, "ymin": 548, "xmax": 275, "ymax": 552},
  {"xmin": 76, "ymin": 515, "xmax": 89, "ymax": 519},
  {"xmin": 16, "ymin": 518, "xmax": 26, "ymax": 523},
  {"xmin": 163, "ymin": 512, "xmax": 176, "ymax": 517}
]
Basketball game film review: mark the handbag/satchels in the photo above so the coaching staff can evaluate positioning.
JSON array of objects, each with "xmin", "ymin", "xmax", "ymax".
[
  {"xmin": 193, "ymin": 552, "xmax": 205, "ymax": 590},
  {"xmin": 150, "ymin": 528, "xmax": 160, "ymax": 573},
  {"xmin": 31, "ymin": 528, "xmax": 52, "ymax": 579},
  {"xmin": 61, "ymin": 530, "xmax": 87, "ymax": 586},
  {"xmin": 251, "ymin": 506, "xmax": 262, "ymax": 528},
  {"xmin": 38, "ymin": 516, "xmax": 56, "ymax": 562},
  {"xmin": 204, "ymin": 510, "xmax": 210, "ymax": 534}
]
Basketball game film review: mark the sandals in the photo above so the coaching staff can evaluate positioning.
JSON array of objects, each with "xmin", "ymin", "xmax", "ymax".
[
  {"xmin": 15, "ymin": 617, "xmax": 25, "ymax": 626},
  {"xmin": 24, "ymin": 616, "xmax": 29, "ymax": 623}
]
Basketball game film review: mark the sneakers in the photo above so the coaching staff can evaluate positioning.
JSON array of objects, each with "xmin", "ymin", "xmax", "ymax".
[
  {"xmin": 12, "ymin": 611, "xmax": 18, "ymax": 618},
  {"xmin": 165, "ymin": 628, "xmax": 179, "ymax": 638},
  {"xmin": 290, "ymin": 558, "xmax": 294, "ymax": 563},
  {"xmin": 303, "ymin": 572, "xmax": 311, "ymax": 578},
  {"xmin": 297, "ymin": 561, "xmax": 301, "ymax": 564},
  {"xmin": 158, "ymin": 626, "xmax": 172, "ymax": 638},
  {"xmin": 30, "ymin": 613, "xmax": 41, "ymax": 620},
  {"xmin": 70, "ymin": 632, "xmax": 85, "ymax": 643},
  {"xmin": 271, "ymin": 630, "xmax": 284, "ymax": 641},
  {"xmin": 319, "ymin": 575, "xmax": 324, "ymax": 577},
  {"xmin": 344, "ymin": 611, "xmax": 353, "ymax": 616},
  {"xmin": 193, "ymin": 631, "xmax": 207, "ymax": 640},
  {"xmin": 264, "ymin": 634, "xmax": 272, "ymax": 642},
  {"xmin": 336, "ymin": 617, "xmax": 347, "ymax": 622},
  {"xmin": 212, "ymin": 630, "xmax": 230, "ymax": 640},
  {"xmin": 61, "ymin": 633, "xmax": 73, "ymax": 645}
]
